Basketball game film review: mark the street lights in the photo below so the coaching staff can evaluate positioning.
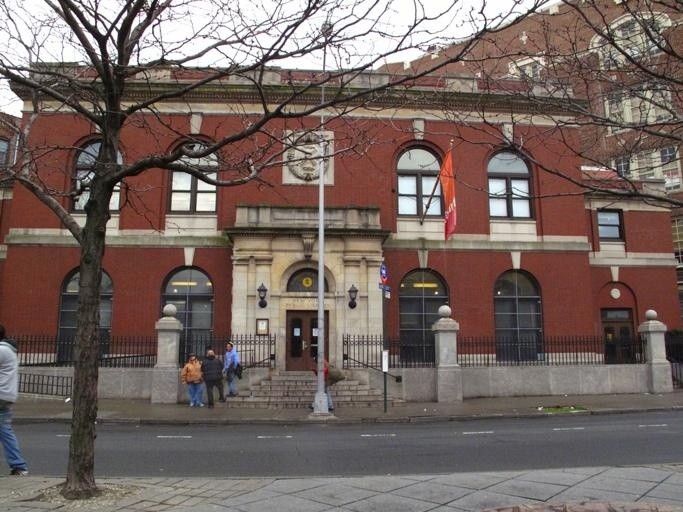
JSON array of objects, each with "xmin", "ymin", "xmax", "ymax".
[{"xmin": 313, "ymin": 22, "xmax": 333, "ymax": 413}]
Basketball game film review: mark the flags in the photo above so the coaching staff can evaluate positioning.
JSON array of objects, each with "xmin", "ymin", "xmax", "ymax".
[{"xmin": 439, "ymin": 148, "xmax": 457, "ymax": 244}]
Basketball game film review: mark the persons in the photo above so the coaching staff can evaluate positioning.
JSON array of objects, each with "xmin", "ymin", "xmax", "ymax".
[
  {"xmin": 180, "ymin": 352, "xmax": 205, "ymax": 407},
  {"xmin": 0, "ymin": 325, "xmax": 29, "ymax": 477},
  {"xmin": 310, "ymin": 360, "xmax": 334, "ymax": 411},
  {"xmin": 223, "ymin": 341, "xmax": 239, "ymax": 397},
  {"xmin": 200, "ymin": 349, "xmax": 226, "ymax": 408}
]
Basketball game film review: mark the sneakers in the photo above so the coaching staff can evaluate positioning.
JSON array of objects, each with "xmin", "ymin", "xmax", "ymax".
[
  {"xmin": 208, "ymin": 404, "xmax": 214, "ymax": 409},
  {"xmin": 9, "ymin": 468, "xmax": 28, "ymax": 476},
  {"xmin": 190, "ymin": 401, "xmax": 204, "ymax": 407},
  {"xmin": 219, "ymin": 399, "xmax": 226, "ymax": 403},
  {"xmin": 226, "ymin": 392, "xmax": 240, "ymax": 397}
]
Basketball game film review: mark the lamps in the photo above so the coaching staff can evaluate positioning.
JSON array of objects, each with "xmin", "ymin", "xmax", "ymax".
[
  {"xmin": 346, "ymin": 283, "xmax": 358, "ymax": 308},
  {"xmin": 256, "ymin": 282, "xmax": 268, "ymax": 308}
]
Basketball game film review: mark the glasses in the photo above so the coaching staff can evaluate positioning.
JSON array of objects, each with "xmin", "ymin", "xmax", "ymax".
[{"xmin": 190, "ymin": 359, "xmax": 195, "ymax": 361}]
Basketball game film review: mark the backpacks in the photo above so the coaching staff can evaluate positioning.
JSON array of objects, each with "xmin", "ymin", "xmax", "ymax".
[{"xmin": 326, "ymin": 365, "xmax": 346, "ymax": 386}]
